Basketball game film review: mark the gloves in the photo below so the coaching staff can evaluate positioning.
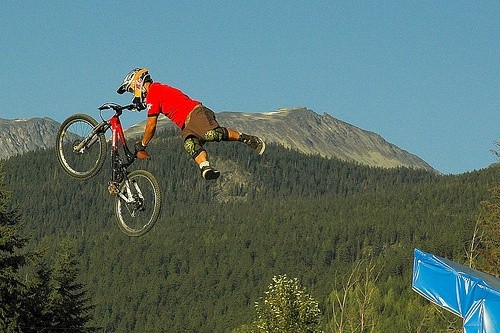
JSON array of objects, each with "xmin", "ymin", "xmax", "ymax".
[
  {"xmin": 130, "ymin": 102, "xmax": 137, "ymax": 112},
  {"xmin": 133, "ymin": 142, "xmax": 146, "ymax": 152}
]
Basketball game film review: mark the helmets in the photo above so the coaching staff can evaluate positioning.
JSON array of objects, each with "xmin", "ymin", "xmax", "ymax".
[{"xmin": 116, "ymin": 66, "xmax": 150, "ymax": 105}]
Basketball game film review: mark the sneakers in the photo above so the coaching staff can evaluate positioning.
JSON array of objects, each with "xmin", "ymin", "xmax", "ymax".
[
  {"xmin": 237, "ymin": 133, "xmax": 265, "ymax": 155},
  {"xmin": 200, "ymin": 166, "xmax": 221, "ymax": 180}
]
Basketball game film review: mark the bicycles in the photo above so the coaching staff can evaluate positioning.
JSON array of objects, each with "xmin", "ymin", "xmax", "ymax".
[{"xmin": 56, "ymin": 103, "xmax": 161, "ymax": 237}]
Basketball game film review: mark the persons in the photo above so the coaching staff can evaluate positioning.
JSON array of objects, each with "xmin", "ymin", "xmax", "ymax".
[{"xmin": 116, "ymin": 67, "xmax": 266, "ymax": 180}]
